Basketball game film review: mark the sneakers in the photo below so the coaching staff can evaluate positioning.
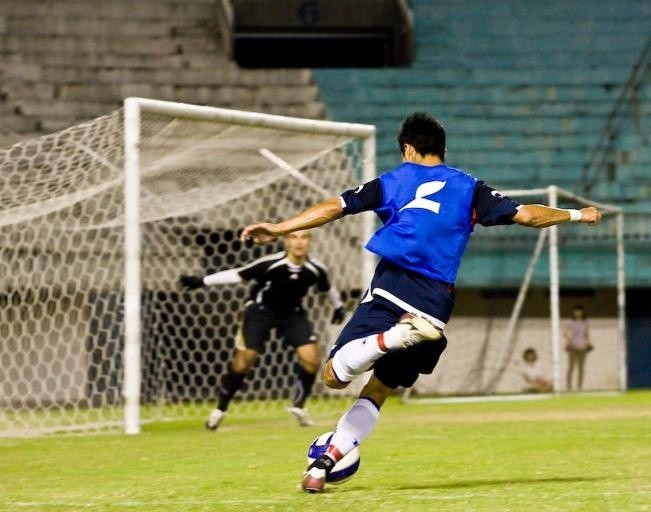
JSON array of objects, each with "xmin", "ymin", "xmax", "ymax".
[
  {"xmin": 397, "ymin": 313, "xmax": 441, "ymax": 348},
  {"xmin": 288, "ymin": 407, "xmax": 314, "ymax": 427},
  {"xmin": 302, "ymin": 468, "xmax": 326, "ymax": 492},
  {"xmin": 207, "ymin": 409, "xmax": 225, "ymax": 429}
]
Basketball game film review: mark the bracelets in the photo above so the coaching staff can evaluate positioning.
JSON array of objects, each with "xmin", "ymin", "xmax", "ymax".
[{"xmin": 567, "ymin": 208, "xmax": 581, "ymax": 222}]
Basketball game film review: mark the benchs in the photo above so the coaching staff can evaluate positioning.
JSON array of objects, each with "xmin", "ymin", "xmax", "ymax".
[{"xmin": 0, "ymin": 18, "xmax": 651, "ymax": 216}]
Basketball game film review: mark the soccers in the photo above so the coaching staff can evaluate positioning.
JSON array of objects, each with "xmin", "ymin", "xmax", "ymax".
[{"xmin": 307, "ymin": 431, "xmax": 362, "ymax": 485}]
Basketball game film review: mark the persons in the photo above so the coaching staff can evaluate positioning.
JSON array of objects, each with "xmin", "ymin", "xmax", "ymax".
[
  {"xmin": 521, "ymin": 348, "xmax": 553, "ymax": 393},
  {"xmin": 563, "ymin": 304, "xmax": 593, "ymax": 392},
  {"xmin": 179, "ymin": 226, "xmax": 346, "ymax": 431},
  {"xmin": 240, "ymin": 110, "xmax": 601, "ymax": 492}
]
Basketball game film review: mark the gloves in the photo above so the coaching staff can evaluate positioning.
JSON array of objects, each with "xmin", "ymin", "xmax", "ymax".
[
  {"xmin": 332, "ymin": 307, "xmax": 346, "ymax": 324},
  {"xmin": 180, "ymin": 275, "xmax": 205, "ymax": 291}
]
